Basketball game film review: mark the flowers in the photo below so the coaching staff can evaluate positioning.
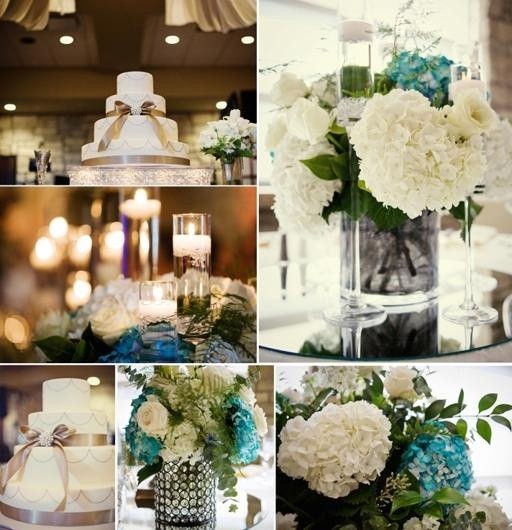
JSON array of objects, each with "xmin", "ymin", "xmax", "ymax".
[
  {"xmin": 122, "ymin": 364, "xmax": 267, "ymax": 466},
  {"xmin": 199, "ymin": 108, "xmax": 257, "ymax": 180},
  {"xmin": 26, "ymin": 269, "xmax": 256, "ymax": 364},
  {"xmin": 276, "ymin": 365, "xmax": 512, "ymax": 530},
  {"xmin": 261, "ymin": 0, "xmax": 502, "ymax": 294}
]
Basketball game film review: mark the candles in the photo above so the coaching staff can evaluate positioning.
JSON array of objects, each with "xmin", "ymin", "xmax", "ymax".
[
  {"xmin": 138, "ymin": 280, "xmax": 176, "ymax": 320},
  {"xmin": 172, "ymin": 211, "xmax": 211, "ymax": 256}
]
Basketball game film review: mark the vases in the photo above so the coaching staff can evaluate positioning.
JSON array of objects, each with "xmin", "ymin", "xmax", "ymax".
[
  {"xmin": 337, "ymin": 129, "xmax": 443, "ymax": 317},
  {"xmin": 150, "ymin": 436, "xmax": 219, "ymax": 530},
  {"xmin": 219, "ymin": 147, "xmax": 244, "ymax": 186}
]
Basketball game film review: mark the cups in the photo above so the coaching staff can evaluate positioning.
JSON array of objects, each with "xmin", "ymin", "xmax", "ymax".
[
  {"xmin": 33, "ymin": 147, "xmax": 51, "ymax": 186},
  {"xmin": 170, "ymin": 211, "xmax": 215, "ymax": 337},
  {"xmin": 137, "ymin": 280, "xmax": 179, "ymax": 347}
]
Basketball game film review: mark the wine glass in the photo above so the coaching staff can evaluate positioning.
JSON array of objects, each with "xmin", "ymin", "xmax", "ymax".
[
  {"xmin": 438, "ymin": 58, "xmax": 500, "ymax": 325},
  {"xmin": 320, "ymin": 18, "xmax": 389, "ymax": 330}
]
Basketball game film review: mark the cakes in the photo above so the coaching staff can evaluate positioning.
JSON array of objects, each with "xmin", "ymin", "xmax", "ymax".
[
  {"xmin": 80, "ymin": 71, "xmax": 192, "ymax": 166},
  {"xmin": 0, "ymin": 377, "xmax": 116, "ymax": 526}
]
